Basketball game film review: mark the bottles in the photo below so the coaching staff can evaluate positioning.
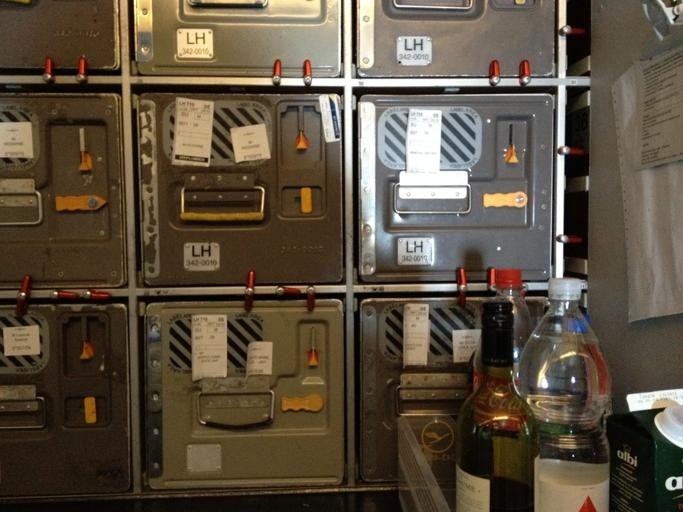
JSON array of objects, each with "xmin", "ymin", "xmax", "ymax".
[
  {"xmin": 473, "ymin": 262, "xmax": 535, "ymax": 387},
  {"xmin": 514, "ymin": 273, "xmax": 615, "ymax": 512},
  {"xmin": 450, "ymin": 297, "xmax": 544, "ymax": 512}
]
